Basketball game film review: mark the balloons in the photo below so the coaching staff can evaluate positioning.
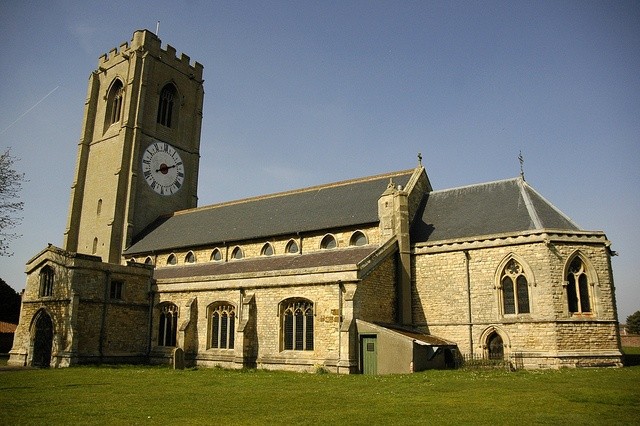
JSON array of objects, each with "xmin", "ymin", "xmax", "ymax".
[{"xmin": 141, "ymin": 142, "xmax": 184, "ymax": 197}]
